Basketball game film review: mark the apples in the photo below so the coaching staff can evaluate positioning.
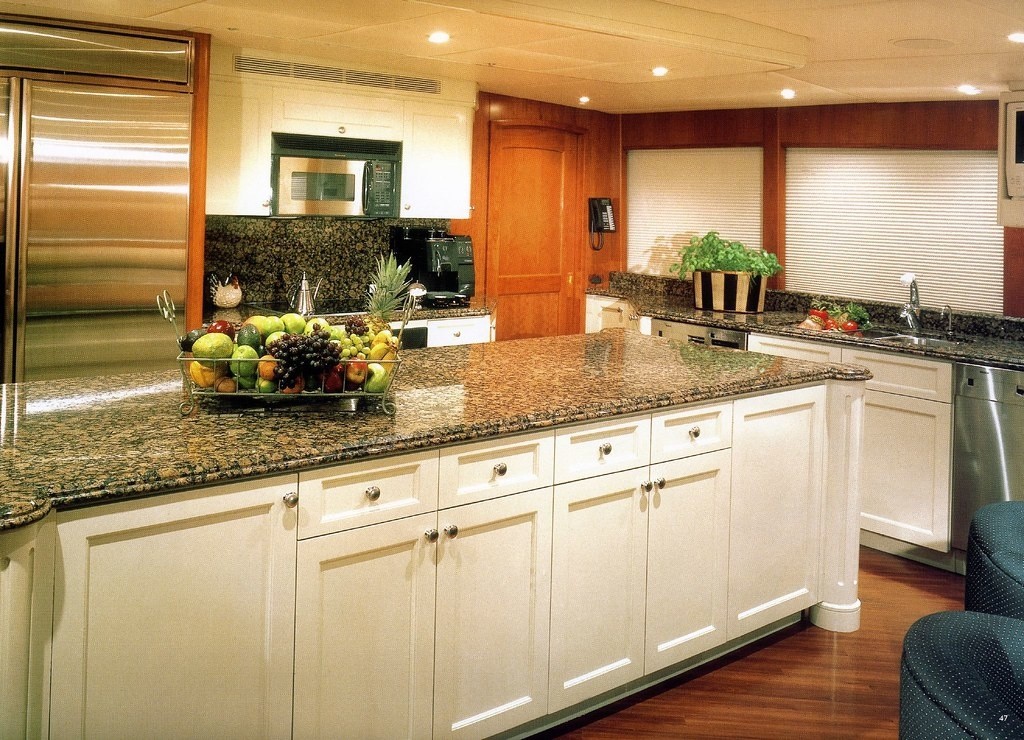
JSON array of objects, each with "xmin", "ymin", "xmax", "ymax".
[
  {"xmin": 191, "ymin": 333, "xmax": 235, "ymax": 368},
  {"xmin": 217, "ymin": 313, "xmax": 389, "ymax": 395},
  {"xmin": 207, "ymin": 319, "xmax": 235, "ymax": 342},
  {"xmin": 190, "ymin": 360, "xmax": 228, "ymax": 388}
]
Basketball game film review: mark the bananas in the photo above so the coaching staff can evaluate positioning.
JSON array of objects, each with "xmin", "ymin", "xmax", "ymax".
[{"xmin": 369, "ymin": 329, "xmax": 400, "ymax": 375}]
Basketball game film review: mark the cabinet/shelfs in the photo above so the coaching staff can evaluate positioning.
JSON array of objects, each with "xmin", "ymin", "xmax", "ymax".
[
  {"xmin": 746, "ymin": 332, "xmax": 956, "ymax": 573},
  {"xmin": 0, "ymin": 332, "xmax": 876, "ymax": 740},
  {"xmin": 427, "ymin": 315, "xmax": 493, "ymax": 349},
  {"xmin": 241, "ymin": 81, "xmax": 473, "ymax": 219},
  {"xmin": 585, "ymin": 295, "xmax": 652, "ymax": 335}
]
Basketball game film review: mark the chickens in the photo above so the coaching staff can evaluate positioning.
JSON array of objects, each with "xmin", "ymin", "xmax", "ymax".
[{"xmin": 215, "ymin": 276, "xmax": 242, "ymax": 308}]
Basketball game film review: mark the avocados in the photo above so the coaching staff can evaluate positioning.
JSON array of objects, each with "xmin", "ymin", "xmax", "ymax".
[
  {"xmin": 237, "ymin": 324, "xmax": 261, "ymax": 350},
  {"xmin": 179, "ymin": 328, "xmax": 207, "ymax": 351}
]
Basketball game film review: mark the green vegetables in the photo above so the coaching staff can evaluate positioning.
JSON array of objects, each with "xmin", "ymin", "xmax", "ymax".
[{"xmin": 809, "ymin": 296, "xmax": 872, "ymax": 328}]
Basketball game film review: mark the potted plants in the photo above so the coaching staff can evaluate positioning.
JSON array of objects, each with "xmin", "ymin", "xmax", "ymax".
[{"xmin": 669, "ymin": 229, "xmax": 784, "ymax": 314}]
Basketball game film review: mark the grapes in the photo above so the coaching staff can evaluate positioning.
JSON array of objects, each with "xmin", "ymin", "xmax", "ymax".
[
  {"xmin": 345, "ymin": 315, "xmax": 370, "ymax": 337},
  {"xmin": 266, "ymin": 333, "xmax": 307, "ymax": 390},
  {"xmin": 302, "ymin": 323, "xmax": 342, "ymax": 371},
  {"xmin": 338, "ymin": 333, "xmax": 371, "ymax": 359}
]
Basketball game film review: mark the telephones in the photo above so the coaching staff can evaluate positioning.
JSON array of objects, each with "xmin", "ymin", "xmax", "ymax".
[{"xmin": 588, "ymin": 197, "xmax": 616, "ymax": 233}]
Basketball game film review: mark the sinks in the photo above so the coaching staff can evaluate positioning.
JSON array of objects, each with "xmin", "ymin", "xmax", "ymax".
[
  {"xmin": 875, "ymin": 336, "xmax": 976, "ymax": 346},
  {"xmin": 818, "ymin": 329, "xmax": 910, "ymax": 339}
]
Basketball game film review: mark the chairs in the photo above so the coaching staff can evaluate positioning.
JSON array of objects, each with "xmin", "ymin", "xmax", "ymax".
[
  {"xmin": 966, "ymin": 501, "xmax": 1024, "ymax": 621},
  {"xmin": 899, "ymin": 611, "xmax": 1024, "ymax": 740}
]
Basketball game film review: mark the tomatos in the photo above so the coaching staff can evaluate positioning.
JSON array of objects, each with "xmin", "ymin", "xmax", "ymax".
[{"xmin": 811, "ymin": 308, "xmax": 858, "ymax": 331}]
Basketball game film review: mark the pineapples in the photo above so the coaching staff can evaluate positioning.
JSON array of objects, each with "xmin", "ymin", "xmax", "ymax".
[{"xmin": 363, "ymin": 249, "xmax": 412, "ymax": 334}]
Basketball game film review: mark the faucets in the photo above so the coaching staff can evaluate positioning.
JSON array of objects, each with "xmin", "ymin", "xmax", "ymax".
[
  {"xmin": 940, "ymin": 304, "xmax": 952, "ymax": 331},
  {"xmin": 899, "ymin": 272, "xmax": 921, "ymax": 332}
]
